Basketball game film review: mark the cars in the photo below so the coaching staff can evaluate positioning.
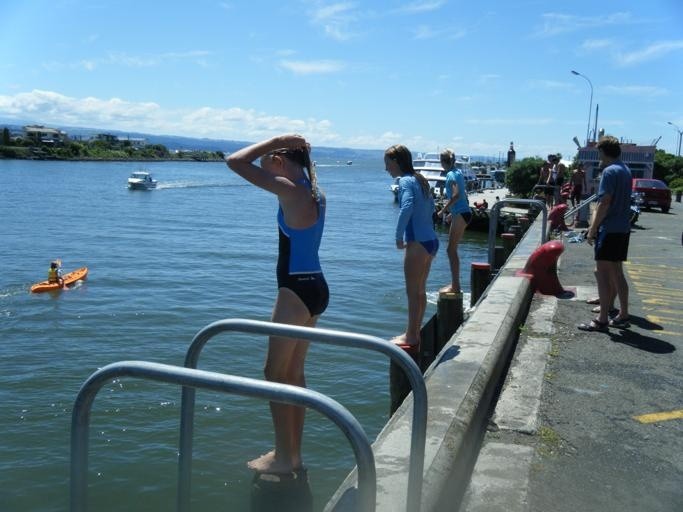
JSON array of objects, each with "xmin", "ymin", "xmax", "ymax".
[{"xmin": 631, "ymin": 178, "xmax": 673, "ymax": 213}]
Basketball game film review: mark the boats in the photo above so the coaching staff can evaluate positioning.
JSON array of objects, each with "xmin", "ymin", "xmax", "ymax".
[
  {"xmin": 30, "ymin": 266, "xmax": 88, "ymax": 293},
  {"xmin": 127, "ymin": 171, "xmax": 157, "ymax": 188},
  {"xmin": 389, "ymin": 152, "xmax": 487, "ymax": 205}
]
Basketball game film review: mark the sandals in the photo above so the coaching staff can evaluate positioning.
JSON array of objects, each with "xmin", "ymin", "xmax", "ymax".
[{"xmin": 577, "ymin": 311, "xmax": 630, "ymax": 331}]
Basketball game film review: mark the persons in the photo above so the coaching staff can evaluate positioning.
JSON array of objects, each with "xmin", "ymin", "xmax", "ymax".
[
  {"xmin": 48, "ymin": 260, "xmax": 69, "ymax": 290},
  {"xmin": 434, "ymin": 148, "xmax": 473, "ymax": 294},
  {"xmin": 380, "ymin": 143, "xmax": 440, "ymax": 348},
  {"xmin": 581, "ymin": 136, "xmax": 634, "ymax": 331},
  {"xmin": 474, "ymin": 194, "xmax": 501, "ymax": 216},
  {"xmin": 531, "ymin": 153, "xmax": 588, "ymax": 210},
  {"xmin": 225, "ymin": 135, "xmax": 330, "ymax": 479}
]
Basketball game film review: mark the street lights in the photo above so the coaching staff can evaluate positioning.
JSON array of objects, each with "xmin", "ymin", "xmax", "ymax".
[{"xmin": 571, "ymin": 70, "xmax": 593, "ymax": 141}]
